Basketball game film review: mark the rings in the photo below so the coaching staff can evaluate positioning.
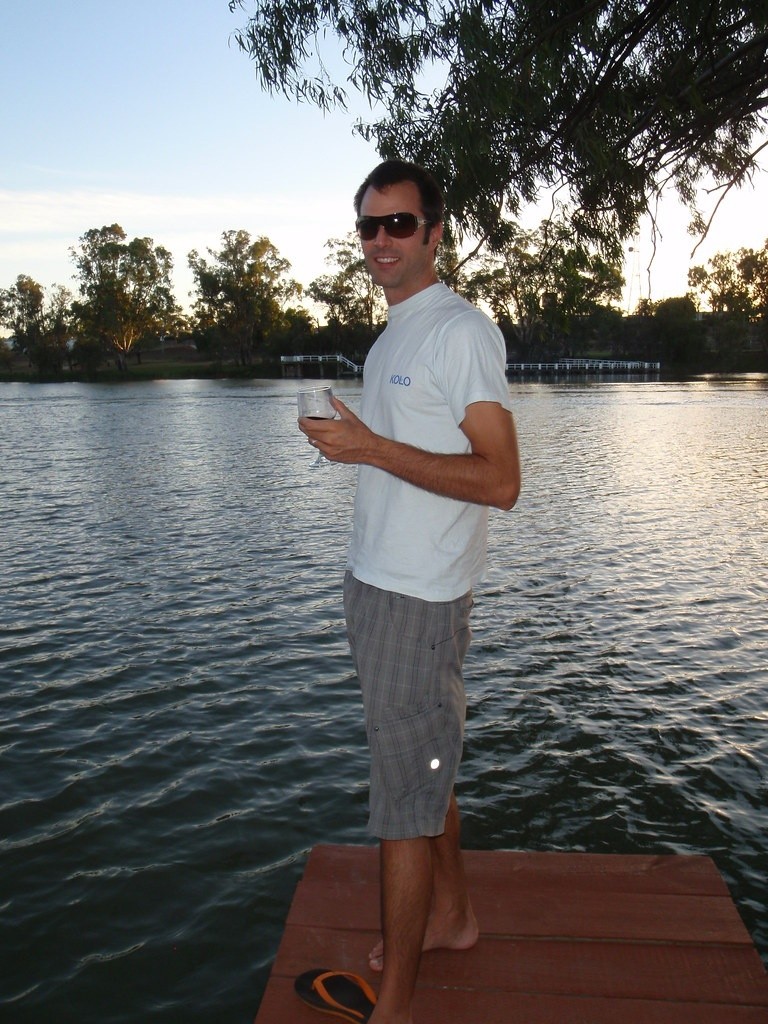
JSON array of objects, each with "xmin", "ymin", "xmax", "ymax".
[{"xmin": 311, "ymin": 440, "xmax": 317, "ymax": 444}]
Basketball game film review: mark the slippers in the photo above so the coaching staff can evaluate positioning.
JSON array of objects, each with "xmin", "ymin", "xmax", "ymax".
[{"xmin": 293, "ymin": 968, "xmax": 377, "ymax": 1024}]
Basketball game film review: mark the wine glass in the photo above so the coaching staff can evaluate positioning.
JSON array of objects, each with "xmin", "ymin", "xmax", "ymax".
[{"xmin": 296, "ymin": 385, "xmax": 337, "ymax": 467}]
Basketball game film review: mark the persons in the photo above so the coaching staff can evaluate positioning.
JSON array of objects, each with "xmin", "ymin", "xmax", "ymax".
[{"xmin": 299, "ymin": 162, "xmax": 520, "ymax": 1023}]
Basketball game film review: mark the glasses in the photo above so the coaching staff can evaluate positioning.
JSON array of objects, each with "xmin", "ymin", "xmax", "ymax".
[{"xmin": 355, "ymin": 212, "xmax": 431, "ymax": 239}]
